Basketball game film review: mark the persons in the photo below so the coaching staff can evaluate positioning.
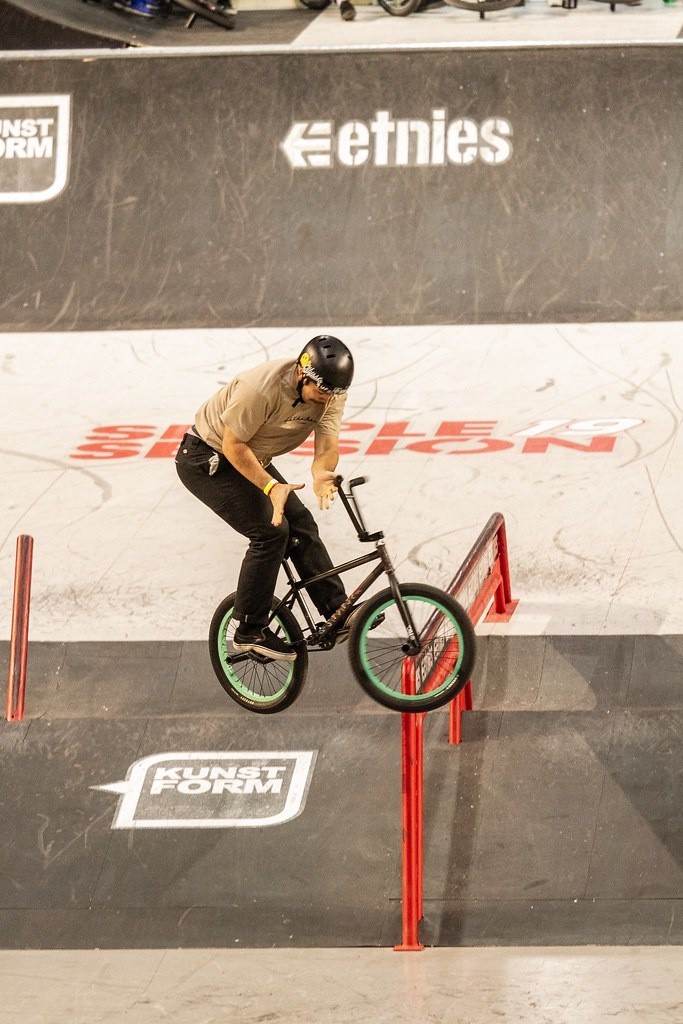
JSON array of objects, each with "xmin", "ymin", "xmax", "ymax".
[{"xmin": 174, "ymin": 335, "xmax": 375, "ymax": 663}]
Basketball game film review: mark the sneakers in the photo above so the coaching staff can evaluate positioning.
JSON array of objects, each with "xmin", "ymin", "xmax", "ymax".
[
  {"xmin": 336, "ymin": 599, "xmax": 368, "ymax": 644},
  {"xmin": 232, "ymin": 626, "xmax": 297, "ymax": 661}
]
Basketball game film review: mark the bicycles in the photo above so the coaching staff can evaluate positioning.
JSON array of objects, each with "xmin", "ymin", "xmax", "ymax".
[
  {"xmin": 173, "ymin": 0, "xmax": 643, "ymax": 30},
  {"xmin": 209, "ymin": 474, "xmax": 477, "ymax": 714}
]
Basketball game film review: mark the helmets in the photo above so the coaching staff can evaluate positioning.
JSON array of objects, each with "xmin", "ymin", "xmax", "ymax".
[{"xmin": 298, "ymin": 335, "xmax": 354, "ymax": 394}]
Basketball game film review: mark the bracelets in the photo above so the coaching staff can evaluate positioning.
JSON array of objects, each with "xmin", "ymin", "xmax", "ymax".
[
  {"xmin": 268, "ymin": 482, "xmax": 280, "ymax": 496},
  {"xmin": 263, "ymin": 479, "xmax": 280, "ymax": 497}
]
